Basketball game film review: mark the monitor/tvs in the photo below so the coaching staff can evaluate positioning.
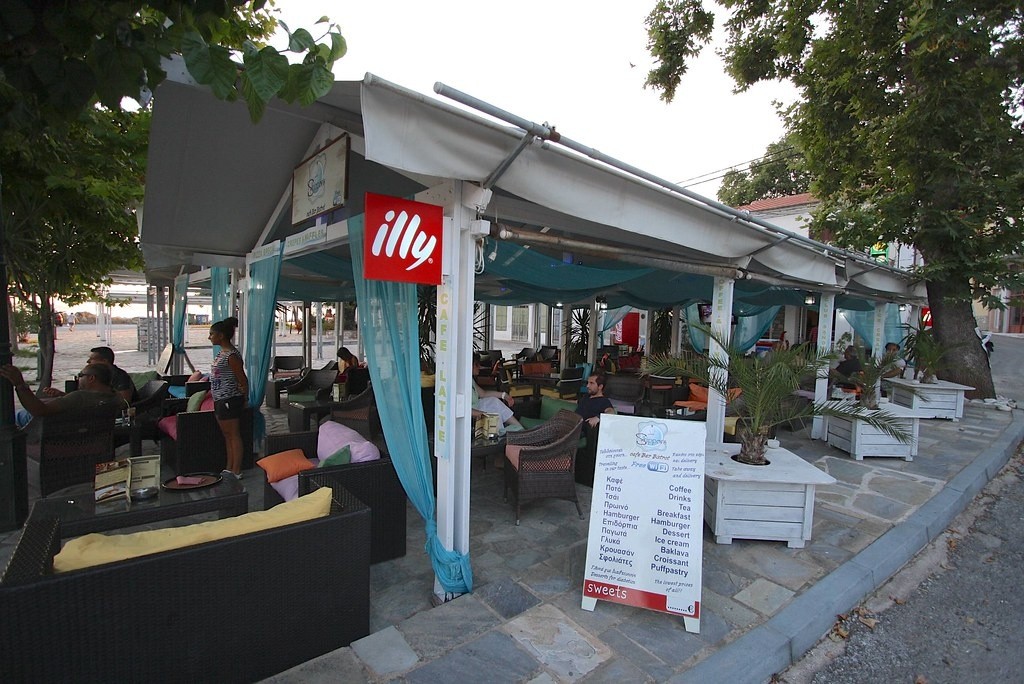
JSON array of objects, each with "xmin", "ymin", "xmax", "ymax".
[{"xmin": 697, "ymin": 304, "xmax": 738, "ymax": 325}]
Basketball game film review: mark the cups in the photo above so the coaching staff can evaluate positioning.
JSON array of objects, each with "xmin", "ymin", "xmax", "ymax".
[
  {"xmin": 122, "ymin": 410, "xmax": 127, "ymax": 427},
  {"xmin": 127, "ymin": 407, "xmax": 136, "ymax": 426},
  {"xmin": 880, "ymin": 397, "xmax": 889, "ymax": 404}
]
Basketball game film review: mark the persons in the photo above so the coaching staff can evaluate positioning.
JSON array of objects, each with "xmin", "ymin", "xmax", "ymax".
[
  {"xmin": 807, "ymin": 327, "xmax": 819, "ymax": 351},
  {"xmin": 470, "ymin": 353, "xmax": 521, "ymax": 426},
  {"xmin": 574, "ymin": 372, "xmax": 614, "ymax": 438},
  {"xmin": 877, "ymin": 343, "xmax": 906, "ymax": 397},
  {"xmin": 209, "ymin": 317, "xmax": 248, "ymax": 480},
  {"xmin": 0, "ymin": 363, "xmax": 112, "ymax": 430},
  {"xmin": 67, "ymin": 313, "xmax": 79, "ymax": 331},
  {"xmin": 316, "ymin": 348, "xmax": 358, "ymax": 399},
  {"xmin": 834, "ymin": 346, "xmax": 864, "ymax": 378},
  {"xmin": 57, "ymin": 313, "xmax": 64, "ymax": 326},
  {"xmin": 42, "ymin": 347, "xmax": 141, "ymax": 401}
]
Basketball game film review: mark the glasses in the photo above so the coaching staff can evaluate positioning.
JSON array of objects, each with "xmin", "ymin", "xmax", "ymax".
[
  {"xmin": 476, "ymin": 362, "xmax": 479, "ymax": 367},
  {"xmin": 79, "ymin": 373, "xmax": 87, "ymax": 377}
]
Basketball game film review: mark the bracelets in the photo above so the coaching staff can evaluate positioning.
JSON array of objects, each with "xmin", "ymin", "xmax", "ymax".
[{"xmin": 501, "ymin": 391, "xmax": 506, "ymax": 400}]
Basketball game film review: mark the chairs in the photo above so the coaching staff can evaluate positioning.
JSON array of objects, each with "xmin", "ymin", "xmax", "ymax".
[
  {"xmin": 420, "ymin": 346, "xmax": 745, "ymax": 526},
  {"xmin": 0, "ymin": 356, "xmax": 406, "ymax": 564}
]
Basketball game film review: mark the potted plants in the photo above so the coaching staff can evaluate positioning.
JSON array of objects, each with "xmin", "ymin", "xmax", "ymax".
[
  {"xmin": 882, "ymin": 314, "xmax": 975, "ymax": 423},
  {"xmin": 824, "ymin": 342, "xmax": 920, "ymax": 462},
  {"xmin": 633, "ymin": 312, "xmax": 918, "ymax": 548}
]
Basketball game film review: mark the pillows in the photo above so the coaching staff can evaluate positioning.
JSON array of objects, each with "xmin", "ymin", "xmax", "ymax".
[
  {"xmin": 256, "ymin": 446, "xmax": 314, "ymax": 483},
  {"xmin": 198, "ymin": 374, "xmax": 209, "ymax": 383},
  {"xmin": 316, "ymin": 444, "xmax": 352, "ymax": 469},
  {"xmin": 269, "ymin": 474, "xmax": 298, "ymax": 502},
  {"xmin": 187, "ymin": 368, "xmax": 201, "ymax": 382},
  {"xmin": 200, "ymin": 389, "xmax": 215, "ymax": 412},
  {"xmin": 186, "ymin": 389, "xmax": 205, "ymax": 412},
  {"xmin": 125, "ymin": 368, "xmax": 157, "ymax": 392}
]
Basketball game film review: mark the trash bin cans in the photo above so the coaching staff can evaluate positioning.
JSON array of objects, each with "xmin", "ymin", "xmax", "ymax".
[
  {"xmin": 196, "ymin": 315, "xmax": 209, "ymax": 325},
  {"xmin": 188, "ymin": 313, "xmax": 196, "ymax": 325}
]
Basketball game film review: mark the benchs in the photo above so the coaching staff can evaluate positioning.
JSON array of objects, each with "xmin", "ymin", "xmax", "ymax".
[{"xmin": 0, "ymin": 473, "xmax": 370, "ymax": 684}]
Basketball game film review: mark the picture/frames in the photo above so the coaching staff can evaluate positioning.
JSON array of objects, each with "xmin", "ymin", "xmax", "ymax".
[{"xmin": 291, "ymin": 131, "xmax": 347, "ymax": 227}]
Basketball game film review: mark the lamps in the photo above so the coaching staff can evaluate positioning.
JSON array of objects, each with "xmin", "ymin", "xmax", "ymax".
[{"xmin": 805, "ymin": 292, "xmax": 816, "ymax": 305}]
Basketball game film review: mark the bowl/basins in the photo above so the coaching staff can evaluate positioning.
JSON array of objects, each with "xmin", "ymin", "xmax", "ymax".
[{"xmin": 767, "ymin": 439, "xmax": 780, "ymax": 449}]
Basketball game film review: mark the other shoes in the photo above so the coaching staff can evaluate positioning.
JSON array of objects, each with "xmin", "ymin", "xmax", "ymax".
[{"xmin": 219, "ymin": 469, "xmax": 245, "ymax": 480}]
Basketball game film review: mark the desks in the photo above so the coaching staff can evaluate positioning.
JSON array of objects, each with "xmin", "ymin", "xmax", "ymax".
[
  {"xmin": 519, "ymin": 372, "xmax": 560, "ymax": 397},
  {"xmin": 23, "ymin": 472, "xmax": 250, "ymax": 521},
  {"xmin": 470, "ymin": 436, "xmax": 506, "ymax": 472}
]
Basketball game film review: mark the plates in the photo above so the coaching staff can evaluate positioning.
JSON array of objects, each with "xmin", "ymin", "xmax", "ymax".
[{"xmin": 161, "ymin": 471, "xmax": 223, "ymax": 491}]
